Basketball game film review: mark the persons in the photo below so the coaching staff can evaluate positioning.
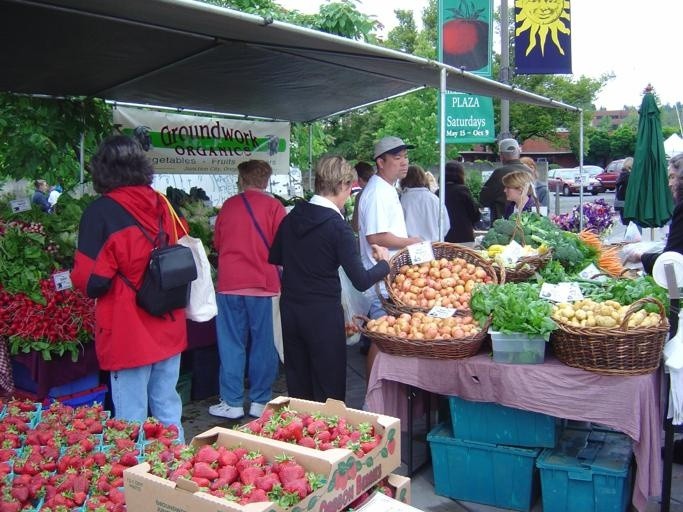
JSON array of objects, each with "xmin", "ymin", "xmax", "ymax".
[
  {"xmin": 68, "ymin": 134, "xmax": 190, "ymax": 450},
  {"xmin": 501, "ymin": 170, "xmax": 536, "ymax": 220},
  {"xmin": 478, "ymin": 138, "xmax": 532, "ymax": 226},
  {"xmin": 206, "ymin": 159, "xmax": 287, "ymax": 420},
  {"xmin": 398, "ymin": 165, "xmax": 450, "ymax": 244},
  {"xmin": 519, "ymin": 156, "xmax": 550, "ymax": 216},
  {"xmin": 357, "ymin": 135, "xmax": 424, "ymax": 392},
  {"xmin": 351, "ymin": 162, "xmax": 375, "ymax": 234},
  {"xmin": 32, "ymin": 179, "xmax": 51, "ymax": 213},
  {"xmin": 624, "ymin": 151, "xmax": 681, "ymax": 277},
  {"xmin": 47, "ymin": 182, "xmax": 63, "ymax": 212},
  {"xmin": 615, "ymin": 157, "xmax": 642, "ymax": 236},
  {"xmin": 268, "ymin": 154, "xmax": 391, "ymax": 404},
  {"xmin": 435, "ymin": 161, "xmax": 481, "ymax": 244}
]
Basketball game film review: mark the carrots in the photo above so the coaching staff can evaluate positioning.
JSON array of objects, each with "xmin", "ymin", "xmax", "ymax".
[{"xmin": 0, "ymin": 266, "xmax": 94, "ymax": 345}]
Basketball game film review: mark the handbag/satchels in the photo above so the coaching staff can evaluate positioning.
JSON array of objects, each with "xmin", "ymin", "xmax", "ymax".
[
  {"xmin": 613, "ymin": 200, "xmax": 626, "ymax": 211},
  {"xmin": 153, "ymin": 186, "xmax": 220, "ymax": 324}
]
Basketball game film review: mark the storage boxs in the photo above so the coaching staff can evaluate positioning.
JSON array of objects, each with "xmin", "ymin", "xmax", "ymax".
[
  {"xmin": 535, "ymin": 428, "xmax": 640, "ymax": 511},
  {"xmin": 486, "ymin": 325, "xmax": 548, "ymax": 367},
  {"xmin": 445, "ymin": 392, "xmax": 562, "ymax": 447},
  {"xmin": 122, "ymin": 394, "xmax": 400, "ymax": 512},
  {"xmin": 10, "ymin": 356, "xmax": 99, "ymax": 396},
  {"xmin": 426, "ymin": 421, "xmax": 541, "ymax": 510},
  {"xmin": 350, "ymin": 472, "xmax": 413, "ymax": 512},
  {"xmin": 43, "ymin": 383, "xmax": 108, "ymax": 413}
]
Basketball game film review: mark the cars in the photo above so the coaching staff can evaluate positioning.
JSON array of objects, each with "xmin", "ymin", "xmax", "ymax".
[
  {"xmin": 471, "ymin": 166, "xmax": 498, "ymax": 233},
  {"xmin": 548, "ymin": 157, "xmax": 633, "ymax": 198}
]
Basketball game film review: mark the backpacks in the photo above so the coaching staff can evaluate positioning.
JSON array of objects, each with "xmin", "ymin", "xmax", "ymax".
[{"xmin": 102, "ymin": 195, "xmax": 197, "ymax": 317}]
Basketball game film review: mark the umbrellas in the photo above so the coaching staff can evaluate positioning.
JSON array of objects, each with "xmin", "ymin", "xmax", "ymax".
[{"xmin": 621, "ymin": 83, "xmax": 675, "ymax": 241}]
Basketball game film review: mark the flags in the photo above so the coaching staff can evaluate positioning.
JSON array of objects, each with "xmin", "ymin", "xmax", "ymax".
[
  {"xmin": 434, "ymin": 0, "xmax": 493, "ymax": 80},
  {"xmin": 437, "ymin": 89, "xmax": 495, "ymax": 146},
  {"xmin": 514, "ymin": 1, "xmax": 573, "ymax": 76}
]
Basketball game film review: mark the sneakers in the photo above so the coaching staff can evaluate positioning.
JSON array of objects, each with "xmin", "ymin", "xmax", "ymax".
[
  {"xmin": 208, "ymin": 401, "xmax": 245, "ymax": 421},
  {"xmin": 248, "ymin": 400, "xmax": 268, "ymax": 418}
]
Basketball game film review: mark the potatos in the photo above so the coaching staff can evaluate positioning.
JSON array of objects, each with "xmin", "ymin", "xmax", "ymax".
[{"xmin": 551, "ymin": 297, "xmax": 662, "ymax": 328}]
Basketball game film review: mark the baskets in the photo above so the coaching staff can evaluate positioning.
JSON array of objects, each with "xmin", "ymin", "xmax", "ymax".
[
  {"xmin": 489, "ymin": 245, "xmax": 558, "ymax": 287},
  {"xmin": 386, "ymin": 239, "xmax": 507, "ymax": 308},
  {"xmin": 372, "ymin": 280, "xmax": 476, "ymax": 319},
  {"xmin": 548, "ymin": 297, "xmax": 672, "ymax": 377},
  {"xmin": 347, "ymin": 309, "xmax": 494, "ymax": 360}
]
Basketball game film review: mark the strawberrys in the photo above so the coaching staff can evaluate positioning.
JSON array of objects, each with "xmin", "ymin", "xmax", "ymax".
[
  {"xmin": 148, "ymin": 405, "xmax": 394, "ymax": 512},
  {"xmin": 1, "ymin": 396, "xmax": 179, "ymax": 512}
]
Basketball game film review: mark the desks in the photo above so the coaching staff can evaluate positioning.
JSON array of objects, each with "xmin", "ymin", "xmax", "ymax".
[
  {"xmin": 0, "ymin": 310, "xmax": 218, "ymax": 413},
  {"xmin": 361, "ymin": 349, "xmax": 675, "ymax": 512}
]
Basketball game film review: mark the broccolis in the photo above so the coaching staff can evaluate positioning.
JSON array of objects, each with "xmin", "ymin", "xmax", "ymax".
[{"xmin": 480, "ymin": 210, "xmax": 584, "ymax": 268}]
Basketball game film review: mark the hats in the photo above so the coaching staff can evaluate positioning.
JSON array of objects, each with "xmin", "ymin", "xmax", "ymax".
[
  {"xmin": 371, "ymin": 135, "xmax": 416, "ymax": 160},
  {"xmin": 499, "ymin": 137, "xmax": 520, "ymax": 154}
]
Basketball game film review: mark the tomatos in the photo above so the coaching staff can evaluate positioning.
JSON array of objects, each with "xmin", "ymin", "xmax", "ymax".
[{"xmin": 443, "ymin": 18, "xmax": 488, "ymax": 70}]
